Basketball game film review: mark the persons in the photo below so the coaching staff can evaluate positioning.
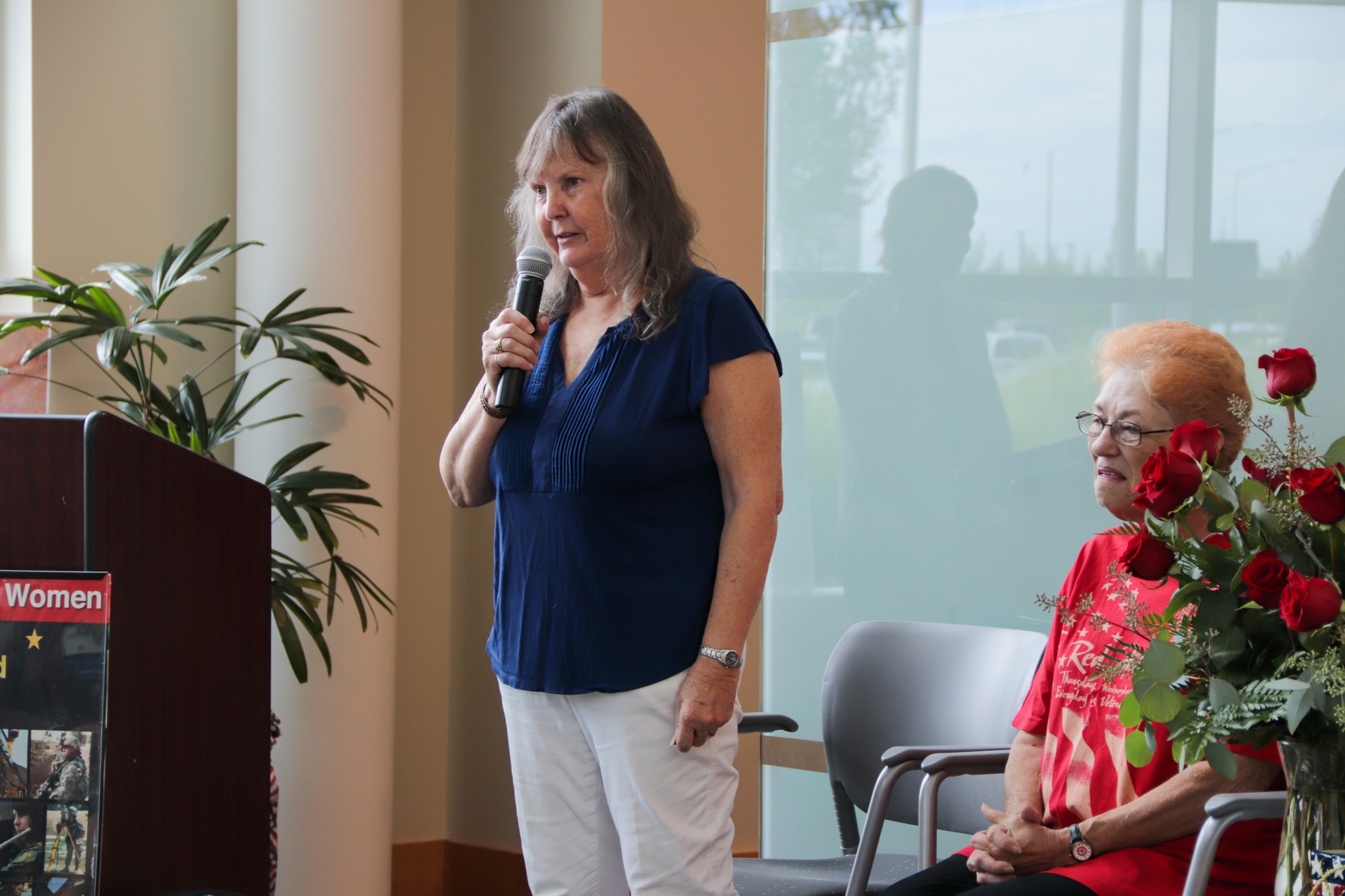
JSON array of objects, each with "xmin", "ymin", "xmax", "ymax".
[
  {"xmin": 439, "ymin": 90, "xmax": 785, "ymax": 896},
  {"xmin": 0, "ymin": 877, "xmax": 25, "ymax": 896},
  {"xmin": 40, "ymin": 737, "xmax": 87, "ymax": 801},
  {"xmin": 0, "ymin": 802, "xmax": 42, "ymax": 872},
  {"xmin": 860, "ymin": 320, "xmax": 1283, "ymax": 896},
  {"xmin": 56, "ymin": 806, "xmax": 85, "ymax": 873}
]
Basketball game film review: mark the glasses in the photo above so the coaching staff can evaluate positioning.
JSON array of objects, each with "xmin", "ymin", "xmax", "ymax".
[{"xmin": 1075, "ymin": 411, "xmax": 1227, "ymax": 446}]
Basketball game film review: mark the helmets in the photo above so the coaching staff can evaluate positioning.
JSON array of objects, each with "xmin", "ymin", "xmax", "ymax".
[
  {"xmin": 63, "ymin": 736, "xmax": 81, "ymax": 751},
  {"xmin": 68, "ymin": 806, "xmax": 77, "ymax": 816}
]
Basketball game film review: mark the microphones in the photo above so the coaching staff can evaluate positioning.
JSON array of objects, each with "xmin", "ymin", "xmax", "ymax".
[{"xmin": 494, "ymin": 246, "xmax": 553, "ymax": 416}]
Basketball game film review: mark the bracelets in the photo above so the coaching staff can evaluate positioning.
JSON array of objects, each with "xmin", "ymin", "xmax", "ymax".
[{"xmin": 480, "ymin": 382, "xmax": 509, "ymax": 419}]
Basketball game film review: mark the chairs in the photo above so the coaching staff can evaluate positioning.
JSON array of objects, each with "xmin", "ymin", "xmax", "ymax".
[{"xmin": 718, "ymin": 620, "xmax": 1289, "ymax": 894}]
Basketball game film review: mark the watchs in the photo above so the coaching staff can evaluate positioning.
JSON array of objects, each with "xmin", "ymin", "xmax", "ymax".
[
  {"xmin": 698, "ymin": 645, "xmax": 743, "ymax": 669},
  {"xmin": 1068, "ymin": 823, "xmax": 1092, "ymax": 863}
]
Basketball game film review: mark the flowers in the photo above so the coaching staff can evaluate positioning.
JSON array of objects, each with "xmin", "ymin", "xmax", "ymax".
[{"xmin": 1107, "ymin": 340, "xmax": 1345, "ymax": 896}]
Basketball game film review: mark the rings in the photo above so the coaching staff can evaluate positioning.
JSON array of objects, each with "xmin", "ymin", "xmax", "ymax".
[
  {"xmin": 708, "ymin": 733, "xmax": 716, "ymax": 738},
  {"xmin": 495, "ymin": 338, "xmax": 503, "ymax": 353},
  {"xmin": 495, "ymin": 354, "xmax": 500, "ymax": 366},
  {"xmin": 695, "ymin": 734, "xmax": 709, "ymax": 740}
]
